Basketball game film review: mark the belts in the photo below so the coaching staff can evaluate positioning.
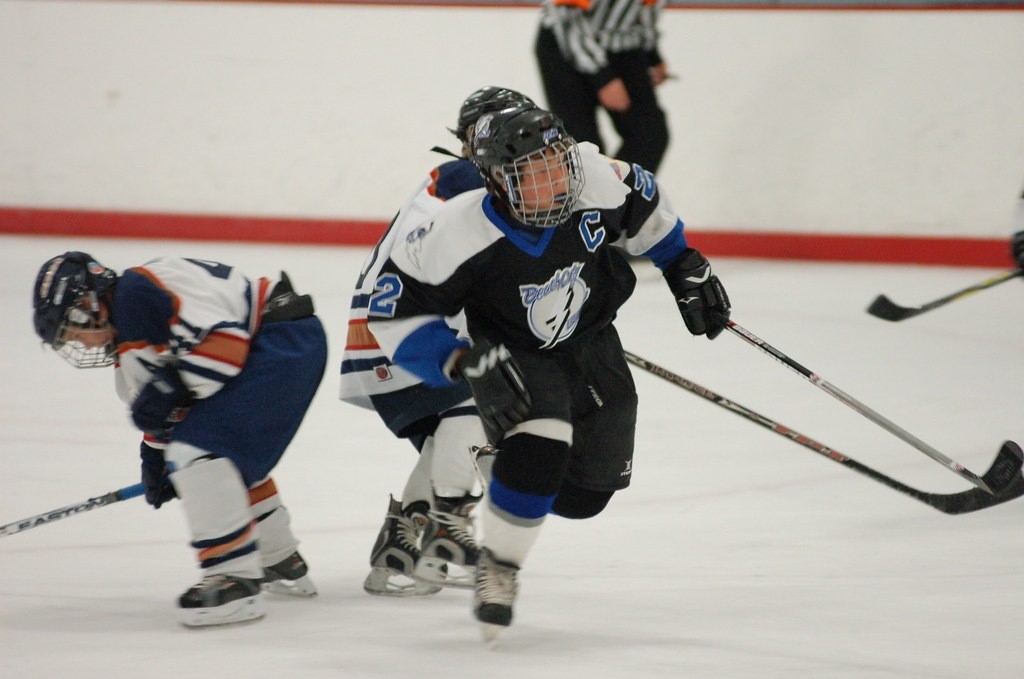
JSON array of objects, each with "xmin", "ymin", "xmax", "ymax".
[{"xmin": 262, "ymin": 295, "xmax": 314, "ymax": 321}]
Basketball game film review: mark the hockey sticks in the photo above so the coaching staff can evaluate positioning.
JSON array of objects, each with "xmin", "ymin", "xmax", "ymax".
[
  {"xmin": 860, "ymin": 266, "xmax": 1024, "ymax": 326},
  {"xmin": 719, "ymin": 314, "xmax": 1024, "ymax": 500},
  {"xmin": 616, "ymin": 343, "xmax": 1024, "ymax": 522},
  {"xmin": 0, "ymin": 481, "xmax": 145, "ymax": 541}
]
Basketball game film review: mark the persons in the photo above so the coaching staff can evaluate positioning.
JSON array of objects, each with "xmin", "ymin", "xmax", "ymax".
[
  {"xmin": 338, "ymin": 86, "xmax": 729, "ymax": 649},
  {"xmin": 533, "ymin": 0, "xmax": 670, "ymax": 265},
  {"xmin": 34, "ymin": 252, "xmax": 330, "ymax": 626}
]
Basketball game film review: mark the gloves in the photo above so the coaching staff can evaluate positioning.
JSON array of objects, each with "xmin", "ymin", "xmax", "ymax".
[
  {"xmin": 139, "ymin": 440, "xmax": 177, "ymax": 509},
  {"xmin": 131, "ymin": 363, "xmax": 192, "ymax": 435},
  {"xmin": 663, "ymin": 251, "xmax": 731, "ymax": 339},
  {"xmin": 456, "ymin": 338, "xmax": 533, "ymax": 432}
]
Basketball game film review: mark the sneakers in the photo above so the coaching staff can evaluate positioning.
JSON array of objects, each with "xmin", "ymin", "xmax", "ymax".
[
  {"xmin": 259, "ymin": 551, "xmax": 318, "ymax": 598},
  {"xmin": 467, "ymin": 445, "xmax": 500, "ymax": 495},
  {"xmin": 179, "ymin": 575, "xmax": 269, "ymax": 628},
  {"xmin": 415, "ymin": 487, "xmax": 484, "ymax": 588},
  {"xmin": 475, "ymin": 546, "xmax": 521, "ymax": 643},
  {"xmin": 364, "ymin": 493, "xmax": 442, "ymax": 597}
]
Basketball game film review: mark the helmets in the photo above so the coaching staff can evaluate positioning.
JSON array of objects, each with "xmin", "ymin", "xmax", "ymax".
[
  {"xmin": 34, "ymin": 252, "xmax": 116, "ymax": 347},
  {"xmin": 479, "ymin": 102, "xmax": 567, "ymax": 183},
  {"xmin": 447, "ymin": 85, "xmax": 536, "ymax": 143}
]
